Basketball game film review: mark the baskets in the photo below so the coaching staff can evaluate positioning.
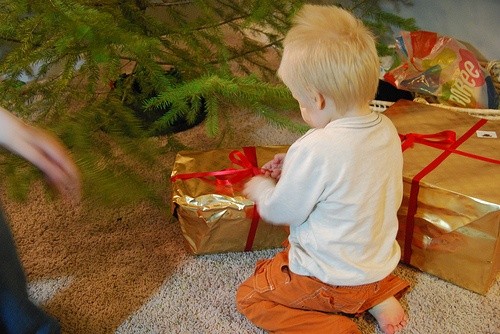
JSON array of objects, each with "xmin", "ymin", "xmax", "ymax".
[{"xmin": 415, "ymin": 58, "xmax": 500, "ymax": 119}]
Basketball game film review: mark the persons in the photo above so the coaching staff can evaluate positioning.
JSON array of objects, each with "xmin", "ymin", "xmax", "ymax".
[{"xmin": 237, "ymin": 4, "xmax": 412, "ymax": 334}]
